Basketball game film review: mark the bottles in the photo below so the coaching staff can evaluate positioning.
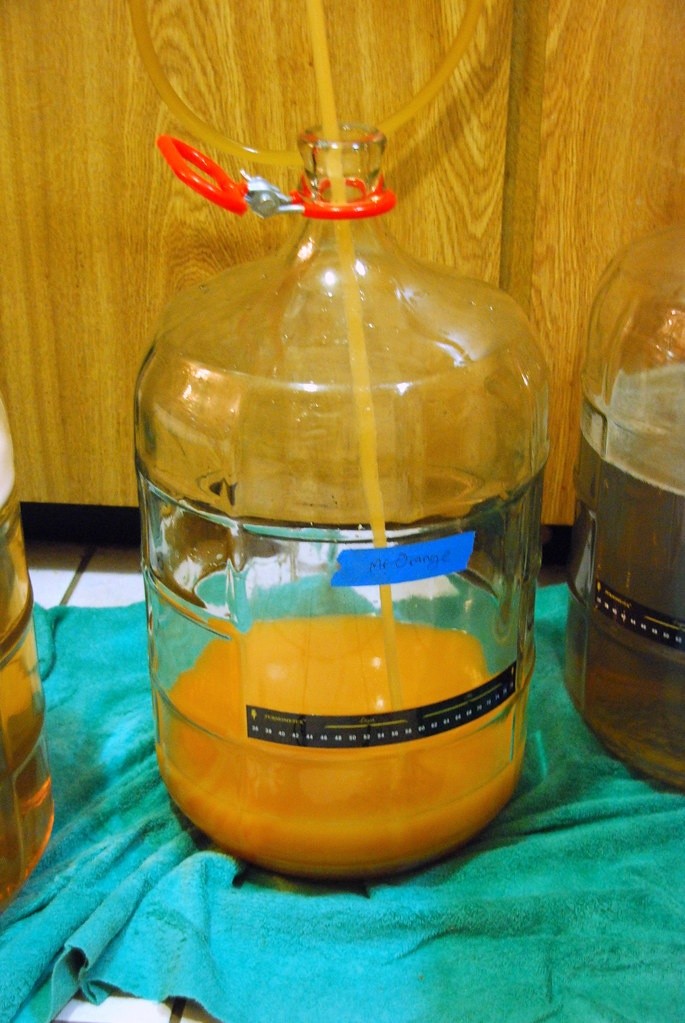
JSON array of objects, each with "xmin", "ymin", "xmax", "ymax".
[
  {"xmin": 132, "ymin": 126, "xmax": 552, "ymax": 880},
  {"xmin": 563, "ymin": 221, "xmax": 685, "ymax": 793},
  {"xmin": 0, "ymin": 397, "xmax": 57, "ymax": 915}
]
